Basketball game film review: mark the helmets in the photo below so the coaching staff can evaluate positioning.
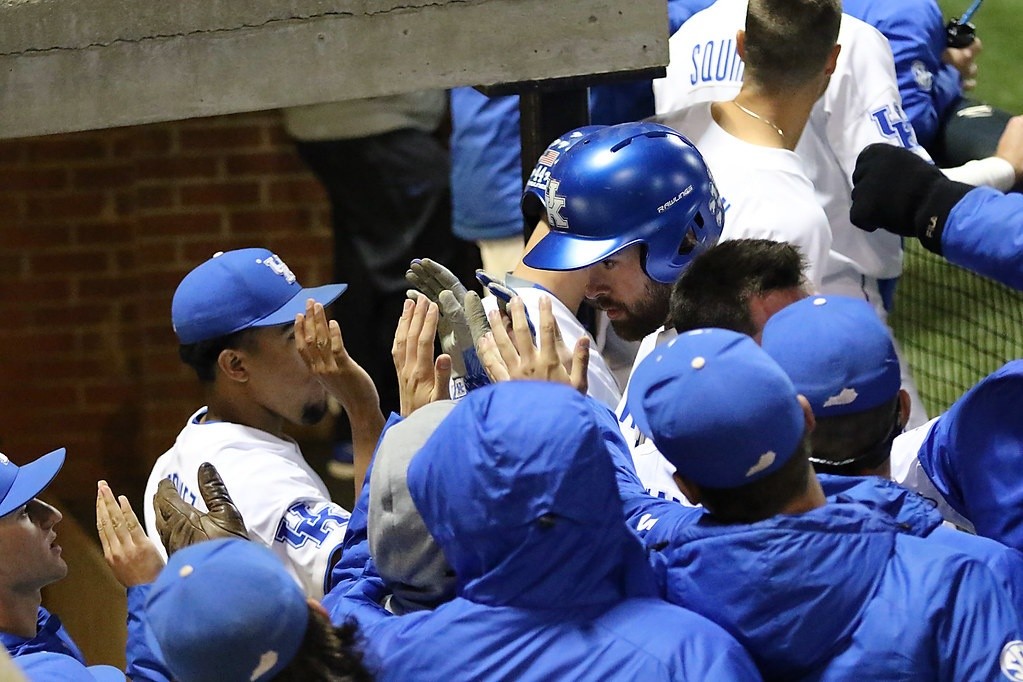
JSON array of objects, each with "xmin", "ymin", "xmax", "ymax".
[
  {"xmin": 522, "ymin": 123, "xmax": 609, "ymax": 223},
  {"xmin": 521, "ymin": 122, "xmax": 724, "ymax": 285}
]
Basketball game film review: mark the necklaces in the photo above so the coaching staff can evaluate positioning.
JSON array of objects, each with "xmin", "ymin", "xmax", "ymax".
[{"xmin": 734, "ymin": 101, "xmax": 788, "ymax": 148}]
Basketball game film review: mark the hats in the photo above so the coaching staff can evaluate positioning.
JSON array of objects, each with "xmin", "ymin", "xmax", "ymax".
[
  {"xmin": 144, "ymin": 538, "xmax": 310, "ymax": 682},
  {"xmin": 171, "ymin": 248, "xmax": 348, "ymax": 345},
  {"xmin": 627, "ymin": 328, "xmax": 804, "ymax": 486},
  {"xmin": 0, "ymin": 447, "xmax": 67, "ymax": 517},
  {"xmin": 762, "ymin": 295, "xmax": 902, "ymax": 415}
]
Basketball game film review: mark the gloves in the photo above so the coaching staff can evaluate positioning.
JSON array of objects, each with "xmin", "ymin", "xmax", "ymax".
[
  {"xmin": 849, "ymin": 143, "xmax": 977, "ymax": 257},
  {"xmin": 405, "ymin": 257, "xmax": 492, "ymax": 401},
  {"xmin": 152, "ymin": 462, "xmax": 250, "ymax": 558},
  {"xmin": 464, "ymin": 269, "xmax": 537, "ymax": 350}
]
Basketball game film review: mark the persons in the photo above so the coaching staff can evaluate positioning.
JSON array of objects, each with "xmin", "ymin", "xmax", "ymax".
[
  {"xmin": 0, "ymin": 448, "xmax": 170, "ymax": 682},
  {"xmin": 323, "ymin": 294, "xmax": 762, "ymax": 682},
  {"xmin": 292, "ymin": 0, "xmax": 1023, "ymax": 682},
  {"xmin": 145, "ymin": 461, "xmax": 376, "ymax": 682},
  {"xmin": 144, "ymin": 248, "xmax": 385, "ymax": 601}
]
